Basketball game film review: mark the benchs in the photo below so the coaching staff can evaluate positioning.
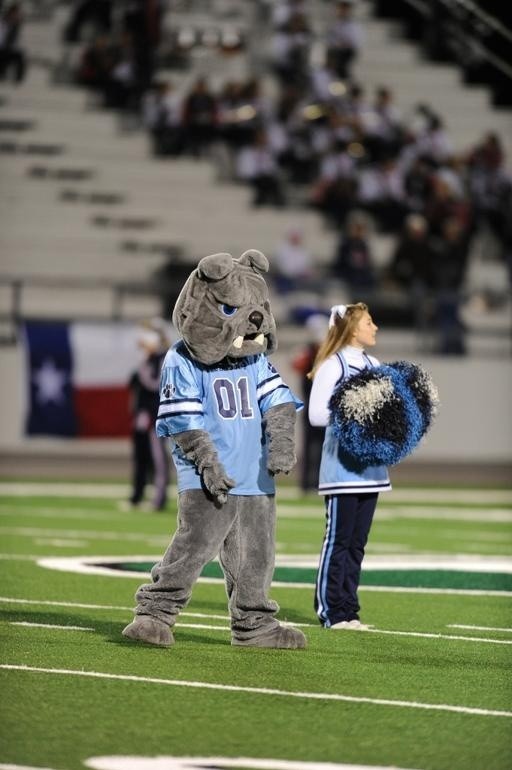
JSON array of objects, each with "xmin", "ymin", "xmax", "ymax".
[{"xmin": 0, "ymin": 0, "xmax": 512, "ymax": 329}]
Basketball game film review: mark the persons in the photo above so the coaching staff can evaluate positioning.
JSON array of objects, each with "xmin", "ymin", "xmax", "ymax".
[
  {"xmin": 290, "ymin": 307, "xmax": 332, "ymax": 492},
  {"xmin": 303, "ymin": 299, "xmax": 395, "ymax": 637},
  {"xmin": 0, "ymin": 1, "xmax": 510, "ymax": 358},
  {"xmin": 122, "ymin": 315, "xmax": 173, "ymax": 515}
]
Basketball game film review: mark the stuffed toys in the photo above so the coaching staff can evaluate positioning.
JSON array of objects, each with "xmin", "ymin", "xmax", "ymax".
[{"xmin": 120, "ymin": 247, "xmax": 310, "ymax": 654}]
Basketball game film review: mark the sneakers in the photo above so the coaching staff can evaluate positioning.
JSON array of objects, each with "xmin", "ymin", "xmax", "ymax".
[{"xmin": 328, "ymin": 617, "xmax": 371, "ymax": 632}]
